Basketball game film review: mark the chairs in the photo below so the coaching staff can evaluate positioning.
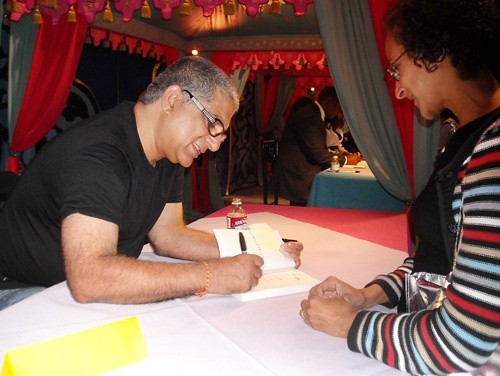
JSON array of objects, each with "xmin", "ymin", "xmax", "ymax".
[{"xmin": 263, "ymin": 138, "xmax": 296, "ymax": 203}]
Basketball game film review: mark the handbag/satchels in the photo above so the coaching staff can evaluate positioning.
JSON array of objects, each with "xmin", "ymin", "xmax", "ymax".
[{"xmin": 404, "ymin": 272, "xmax": 500, "ymax": 376}]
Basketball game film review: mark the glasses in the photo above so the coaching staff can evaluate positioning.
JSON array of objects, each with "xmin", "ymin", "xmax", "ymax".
[
  {"xmin": 387, "ymin": 49, "xmax": 408, "ymax": 81},
  {"xmin": 182, "ymin": 89, "xmax": 226, "ymax": 137}
]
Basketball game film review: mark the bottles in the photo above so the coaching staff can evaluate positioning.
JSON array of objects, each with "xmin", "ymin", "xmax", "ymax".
[
  {"xmin": 226, "ymin": 197, "xmax": 248, "ymax": 230},
  {"xmin": 330, "ymin": 156, "xmax": 340, "ymax": 173}
]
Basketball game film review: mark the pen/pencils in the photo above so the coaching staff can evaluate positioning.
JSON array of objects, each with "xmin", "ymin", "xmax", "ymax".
[
  {"xmin": 239, "ymin": 232, "xmax": 247, "ymax": 254},
  {"xmin": 339, "ymin": 171, "xmax": 360, "ymax": 173}
]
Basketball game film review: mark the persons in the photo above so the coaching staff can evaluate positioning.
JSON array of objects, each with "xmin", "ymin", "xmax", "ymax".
[
  {"xmin": 0, "ymin": 57, "xmax": 303, "ymax": 309},
  {"xmin": 300, "ymin": 0, "xmax": 500, "ymax": 376},
  {"xmin": 275, "ymin": 86, "xmax": 360, "ymax": 207}
]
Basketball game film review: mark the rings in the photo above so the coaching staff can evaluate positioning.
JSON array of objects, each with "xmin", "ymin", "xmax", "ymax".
[{"xmin": 301, "ymin": 310, "xmax": 306, "ymax": 319}]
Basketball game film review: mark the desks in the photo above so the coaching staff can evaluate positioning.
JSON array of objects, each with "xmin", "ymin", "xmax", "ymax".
[
  {"xmin": 306, "ymin": 161, "xmax": 406, "ymax": 212},
  {"xmin": 0, "ymin": 204, "xmax": 411, "ymax": 376}
]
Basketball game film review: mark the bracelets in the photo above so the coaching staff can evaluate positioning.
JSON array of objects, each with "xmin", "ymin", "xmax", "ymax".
[{"xmin": 196, "ymin": 261, "xmax": 210, "ymax": 296}]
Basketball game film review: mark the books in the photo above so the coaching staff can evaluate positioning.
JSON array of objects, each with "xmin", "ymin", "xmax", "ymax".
[{"xmin": 214, "ymin": 224, "xmax": 320, "ymax": 303}]
